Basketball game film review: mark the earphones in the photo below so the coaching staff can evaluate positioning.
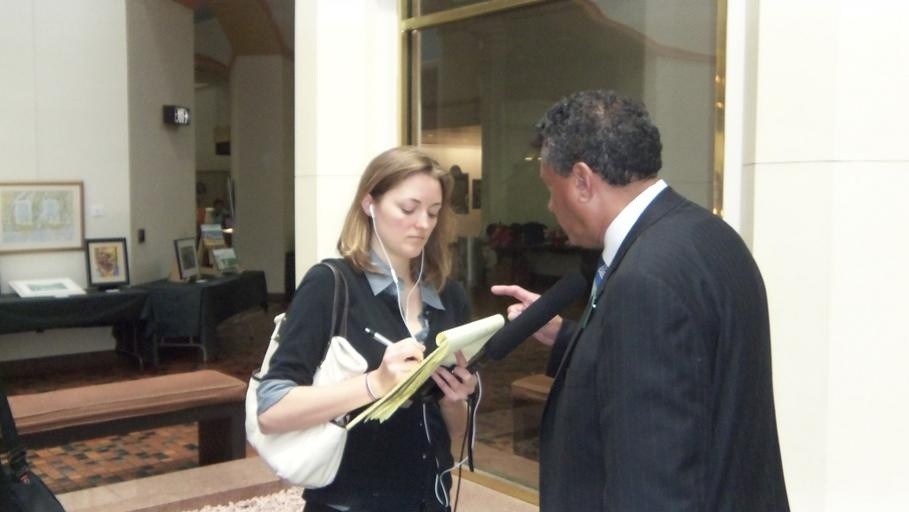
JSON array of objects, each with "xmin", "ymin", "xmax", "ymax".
[{"xmin": 367, "ymin": 202, "xmax": 376, "ymax": 216}]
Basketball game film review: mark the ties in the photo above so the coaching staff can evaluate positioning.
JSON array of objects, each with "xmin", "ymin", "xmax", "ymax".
[{"xmin": 586, "ymin": 261, "xmax": 611, "ymax": 317}]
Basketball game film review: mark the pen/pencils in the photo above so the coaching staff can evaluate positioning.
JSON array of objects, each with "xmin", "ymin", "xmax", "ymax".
[{"xmin": 364, "ymin": 328, "xmax": 391, "ymax": 346}]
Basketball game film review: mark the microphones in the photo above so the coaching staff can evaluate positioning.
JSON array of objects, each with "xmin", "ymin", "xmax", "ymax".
[{"xmin": 437, "ymin": 263, "xmax": 590, "ymax": 411}]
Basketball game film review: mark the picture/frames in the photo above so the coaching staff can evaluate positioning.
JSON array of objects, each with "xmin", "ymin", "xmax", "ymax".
[
  {"xmin": 0, "ymin": 182, "xmax": 85, "ymax": 256},
  {"xmin": 174, "ymin": 237, "xmax": 200, "ymax": 279},
  {"xmin": 84, "ymin": 237, "xmax": 130, "ymax": 288},
  {"xmin": 8, "ymin": 277, "xmax": 86, "ymax": 299}
]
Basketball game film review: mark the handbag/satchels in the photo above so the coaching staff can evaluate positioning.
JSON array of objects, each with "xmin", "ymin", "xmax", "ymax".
[
  {"xmin": 2, "ymin": 416, "xmax": 62, "ymax": 511},
  {"xmin": 244, "ymin": 309, "xmax": 367, "ymax": 491}
]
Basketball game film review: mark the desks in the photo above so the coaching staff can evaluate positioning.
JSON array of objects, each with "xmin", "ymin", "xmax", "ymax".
[
  {"xmin": 490, "ymin": 240, "xmax": 602, "ymax": 309},
  {"xmin": 0, "ymin": 289, "xmax": 149, "ymax": 372},
  {"xmin": 131, "ymin": 270, "xmax": 267, "ymax": 367}
]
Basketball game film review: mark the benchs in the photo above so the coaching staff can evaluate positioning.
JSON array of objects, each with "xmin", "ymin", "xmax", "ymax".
[{"xmin": 6, "ymin": 370, "xmax": 249, "ymax": 467}]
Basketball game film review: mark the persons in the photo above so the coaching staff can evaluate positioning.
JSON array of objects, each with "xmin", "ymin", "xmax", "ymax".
[
  {"xmin": 212, "ymin": 198, "xmax": 229, "ymax": 227},
  {"xmin": 256, "ymin": 144, "xmax": 482, "ymax": 512},
  {"xmin": 491, "ymin": 90, "xmax": 792, "ymax": 512}
]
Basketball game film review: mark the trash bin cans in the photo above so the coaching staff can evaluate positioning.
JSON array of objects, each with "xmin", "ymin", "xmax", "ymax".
[{"xmin": 514, "ymin": 376, "xmax": 552, "ymax": 461}]
